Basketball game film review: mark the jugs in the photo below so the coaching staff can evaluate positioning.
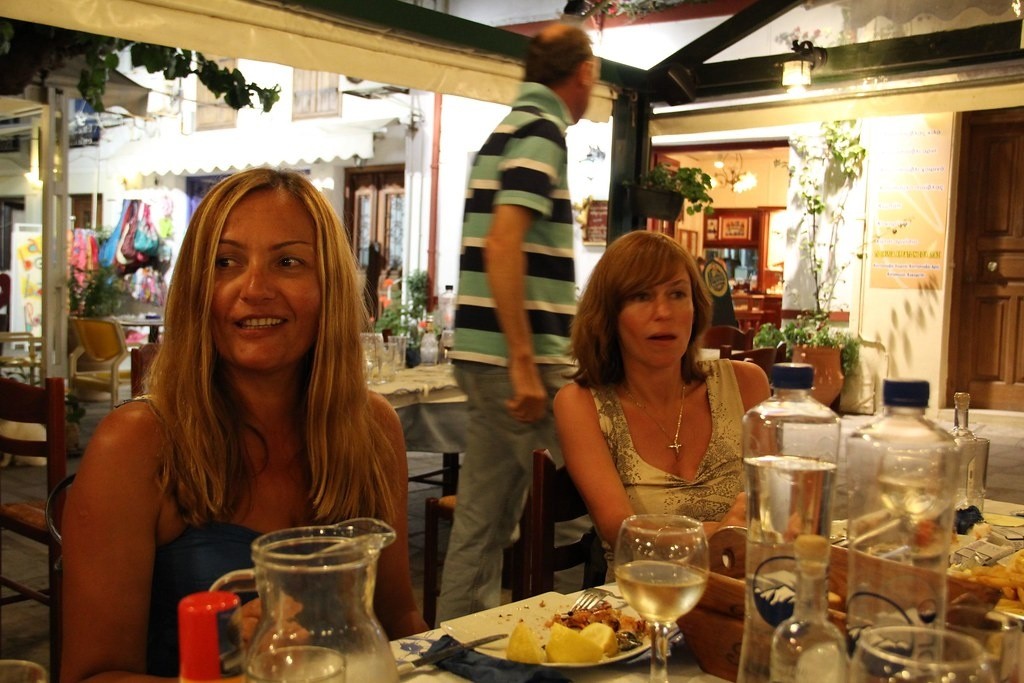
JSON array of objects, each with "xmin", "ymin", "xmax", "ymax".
[{"xmin": 209, "ymin": 516, "xmax": 400, "ymax": 683}]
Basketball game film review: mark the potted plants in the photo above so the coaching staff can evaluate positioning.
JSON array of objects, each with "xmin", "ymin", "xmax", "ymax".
[
  {"xmin": 627, "ymin": 162, "xmax": 713, "ymax": 219},
  {"xmin": 375, "ymin": 269, "xmax": 428, "ymax": 367},
  {"xmin": 753, "ymin": 120, "xmax": 866, "ymax": 406},
  {"xmin": 66, "ymin": 260, "xmax": 130, "ymax": 402}
]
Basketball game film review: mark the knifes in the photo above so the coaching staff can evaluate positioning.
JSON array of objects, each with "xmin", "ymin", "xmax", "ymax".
[{"xmin": 398, "ymin": 634, "xmax": 509, "ymax": 678}]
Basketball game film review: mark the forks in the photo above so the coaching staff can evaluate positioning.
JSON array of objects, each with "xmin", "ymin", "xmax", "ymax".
[{"xmin": 569, "ymin": 587, "xmax": 624, "ymax": 610}]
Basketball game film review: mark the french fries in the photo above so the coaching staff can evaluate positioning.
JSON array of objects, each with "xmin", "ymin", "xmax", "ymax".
[{"xmin": 951, "ymin": 549, "xmax": 1024, "ymax": 613}]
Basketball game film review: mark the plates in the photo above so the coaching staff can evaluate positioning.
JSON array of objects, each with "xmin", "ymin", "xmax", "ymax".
[{"xmin": 440, "ymin": 590, "xmax": 680, "ymax": 667}]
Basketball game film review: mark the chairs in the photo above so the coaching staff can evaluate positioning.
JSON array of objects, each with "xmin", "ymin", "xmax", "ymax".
[
  {"xmin": 0, "ymin": 333, "xmax": 77, "ymax": 683},
  {"xmin": 705, "ymin": 325, "xmax": 787, "ymax": 397},
  {"xmin": 70, "ymin": 315, "xmax": 143, "ymax": 409},
  {"xmin": 419, "ymin": 449, "xmax": 609, "ymax": 632}
]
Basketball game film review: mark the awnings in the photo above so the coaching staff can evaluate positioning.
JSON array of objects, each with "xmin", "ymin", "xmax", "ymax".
[{"xmin": 118, "ymin": 116, "xmax": 401, "ymax": 176}]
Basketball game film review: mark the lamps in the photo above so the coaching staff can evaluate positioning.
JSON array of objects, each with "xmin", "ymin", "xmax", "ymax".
[
  {"xmin": 710, "ymin": 152, "xmax": 757, "ymax": 193},
  {"xmin": 781, "ymin": 40, "xmax": 827, "ymax": 94}
]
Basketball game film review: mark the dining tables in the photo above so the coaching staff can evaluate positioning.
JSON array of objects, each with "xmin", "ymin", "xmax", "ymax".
[{"xmin": 389, "ymin": 499, "xmax": 1024, "ymax": 683}]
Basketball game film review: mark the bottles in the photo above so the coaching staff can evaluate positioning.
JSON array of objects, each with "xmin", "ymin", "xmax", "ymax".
[
  {"xmin": 420, "ymin": 284, "xmax": 454, "ymax": 367},
  {"xmin": 735, "ymin": 364, "xmax": 990, "ymax": 683}
]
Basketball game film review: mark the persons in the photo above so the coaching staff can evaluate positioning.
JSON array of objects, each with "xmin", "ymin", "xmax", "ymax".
[
  {"xmin": 554, "ymin": 230, "xmax": 781, "ymax": 582},
  {"xmin": 434, "ymin": 26, "xmax": 596, "ymax": 625},
  {"xmin": 60, "ymin": 168, "xmax": 428, "ymax": 683}
]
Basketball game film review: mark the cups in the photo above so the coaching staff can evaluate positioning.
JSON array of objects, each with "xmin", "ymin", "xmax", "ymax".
[
  {"xmin": 850, "ymin": 600, "xmax": 1023, "ymax": 683},
  {"xmin": 0, "ymin": 659, "xmax": 45, "ymax": 682},
  {"xmin": 246, "ymin": 645, "xmax": 347, "ymax": 681},
  {"xmin": 360, "ymin": 332, "xmax": 407, "ymax": 386}
]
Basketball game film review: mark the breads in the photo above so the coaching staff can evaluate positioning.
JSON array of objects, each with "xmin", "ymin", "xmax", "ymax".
[{"xmin": 0, "ymin": 502, "xmax": 48, "ymax": 529}]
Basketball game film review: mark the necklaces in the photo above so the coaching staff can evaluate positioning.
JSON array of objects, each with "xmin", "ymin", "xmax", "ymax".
[{"xmin": 626, "ymin": 381, "xmax": 685, "ymax": 453}]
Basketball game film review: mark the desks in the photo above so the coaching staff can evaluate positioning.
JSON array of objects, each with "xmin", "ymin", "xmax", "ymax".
[
  {"xmin": 376, "ymin": 349, "xmax": 740, "ymax": 495},
  {"xmin": 105, "ymin": 313, "xmax": 163, "ymax": 343}
]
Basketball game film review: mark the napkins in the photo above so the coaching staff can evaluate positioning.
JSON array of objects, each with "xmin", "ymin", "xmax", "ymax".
[{"xmin": 419, "ymin": 635, "xmax": 570, "ymax": 682}]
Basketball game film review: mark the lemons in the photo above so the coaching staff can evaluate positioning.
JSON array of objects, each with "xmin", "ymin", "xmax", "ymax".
[{"xmin": 507, "ymin": 622, "xmax": 617, "ymax": 664}]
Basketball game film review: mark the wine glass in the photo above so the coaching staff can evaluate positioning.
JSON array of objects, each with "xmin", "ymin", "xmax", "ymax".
[
  {"xmin": 614, "ymin": 514, "xmax": 710, "ymax": 683},
  {"xmin": 439, "ymin": 329, "xmax": 456, "ymax": 365}
]
